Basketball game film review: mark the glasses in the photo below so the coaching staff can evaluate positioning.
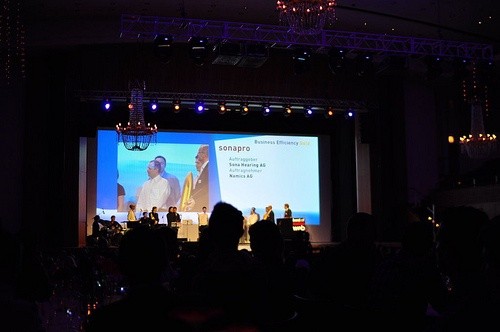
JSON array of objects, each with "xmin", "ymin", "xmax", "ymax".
[
  {"xmin": 148, "ymin": 167, "xmax": 156, "ymax": 170},
  {"xmin": 198, "ymin": 151, "xmax": 204, "ymax": 154}
]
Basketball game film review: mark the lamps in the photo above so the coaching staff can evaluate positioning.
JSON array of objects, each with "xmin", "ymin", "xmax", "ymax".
[
  {"xmin": 323, "ymin": 105, "xmax": 336, "ymax": 120},
  {"xmin": 261, "ymin": 101, "xmax": 271, "ymax": 116},
  {"xmin": 103, "ymin": 97, "xmax": 112, "ymax": 111},
  {"xmin": 329, "ymin": 47, "xmax": 347, "ymax": 70},
  {"xmin": 127, "ymin": 98, "xmax": 133, "ymax": 109},
  {"xmin": 344, "ymin": 106, "xmax": 355, "ymax": 121},
  {"xmin": 281, "ymin": 104, "xmax": 292, "ymax": 118},
  {"xmin": 148, "ymin": 99, "xmax": 157, "ymax": 112},
  {"xmin": 240, "ymin": 98, "xmax": 249, "ymax": 116},
  {"xmin": 186, "ymin": 36, "xmax": 266, "ymax": 67},
  {"xmin": 193, "ymin": 96, "xmax": 204, "ymax": 115},
  {"xmin": 273, "ymin": 0, "xmax": 338, "ymax": 36},
  {"xmin": 114, "ymin": 79, "xmax": 159, "ymax": 151},
  {"xmin": 152, "ymin": 33, "xmax": 172, "ymax": 65},
  {"xmin": 171, "ymin": 95, "xmax": 182, "ymax": 111},
  {"xmin": 288, "ymin": 45, "xmax": 316, "ymax": 66},
  {"xmin": 446, "ymin": 60, "xmax": 500, "ymax": 161},
  {"xmin": 303, "ymin": 105, "xmax": 313, "ymax": 120},
  {"xmin": 217, "ymin": 99, "xmax": 227, "ymax": 115}
]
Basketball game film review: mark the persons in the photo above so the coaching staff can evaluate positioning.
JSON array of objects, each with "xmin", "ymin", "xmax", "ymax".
[
  {"xmin": 108, "ymin": 215, "xmax": 123, "ymax": 229},
  {"xmin": 200, "ymin": 206, "xmax": 210, "ymax": 222},
  {"xmin": 186, "ymin": 145, "xmax": 208, "ymax": 211},
  {"xmin": 128, "ymin": 205, "xmax": 137, "ymax": 220},
  {"xmin": 138, "ymin": 212, "xmax": 150, "ymax": 224},
  {"xmin": 195, "ymin": 154, "xmax": 206, "ymax": 171},
  {"xmin": 149, "ymin": 207, "xmax": 159, "ymax": 224},
  {"xmin": 138, "ymin": 160, "xmax": 171, "ymax": 213},
  {"xmin": 248, "ymin": 207, "xmax": 259, "ymax": 224},
  {"xmin": 284, "ymin": 203, "xmax": 293, "ymax": 218},
  {"xmin": 0, "ymin": 234, "xmax": 45, "ymax": 332},
  {"xmin": 92, "ymin": 215, "xmax": 100, "ymax": 235},
  {"xmin": 117, "ymin": 170, "xmax": 125, "ymax": 212},
  {"xmin": 184, "ymin": 201, "xmax": 500, "ymax": 332},
  {"xmin": 86, "ymin": 228, "xmax": 207, "ymax": 332},
  {"xmin": 167, "ymin": 206, "xmax": 181, "ymax": 226},
  {"xmin": 153, "ymin": 156, "xmax": 181, "ymax": 205},
  {"xmin": 263, "ymin": 205, "xmax": 274, "ymax": 222}
]
